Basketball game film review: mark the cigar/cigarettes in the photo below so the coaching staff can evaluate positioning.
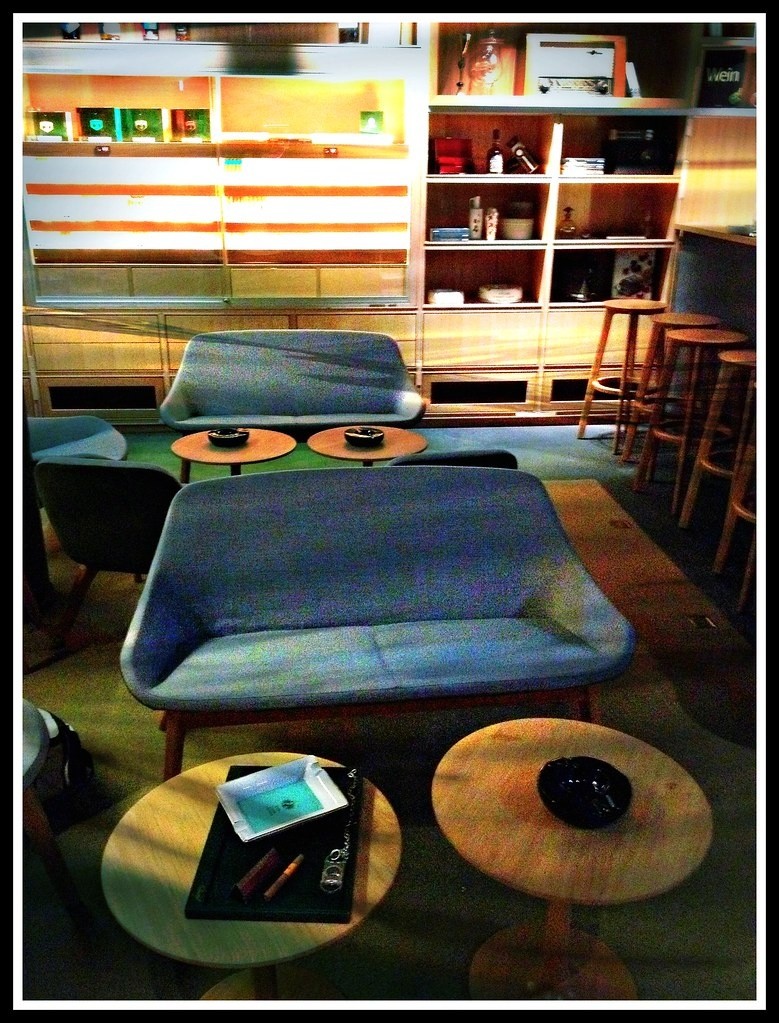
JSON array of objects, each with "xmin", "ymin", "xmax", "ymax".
[{"xmin": 263, "ymin": 853, "xmax": 304, "ymax": 898}]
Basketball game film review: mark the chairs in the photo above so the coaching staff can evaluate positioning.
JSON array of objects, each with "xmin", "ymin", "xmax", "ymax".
[
  {"xmin": 35, "ymin": 456, "xmax": 183, "ymax": 658},
  {"xmin": 28, "ymin": 415, "xmax": 130, "ymax": 507}
]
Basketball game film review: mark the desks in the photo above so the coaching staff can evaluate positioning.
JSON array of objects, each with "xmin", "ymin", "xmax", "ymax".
[
  {"xmin": 100, "ymin": 752, "xmax": 404, "ymax": 1001},
  {"xmin": 171, "ymin": 428, "xmax": 296, "ymax": 476},
  {"xmin": 307, "ymin": 426, "xmax": 427, "ymax": 466},
  {"xmin": 429, "ymin": 716, "xmax": 714, "ymax": 1000}
]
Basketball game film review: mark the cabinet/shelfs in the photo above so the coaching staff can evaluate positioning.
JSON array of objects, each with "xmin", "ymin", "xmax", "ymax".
[{"xmin": 23, "ymin": 22, "xmax": 757, "ymax": 429}]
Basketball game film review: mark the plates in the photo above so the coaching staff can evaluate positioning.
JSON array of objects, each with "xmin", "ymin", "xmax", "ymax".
[
  {"xmin": 477, "ymin": 283, "xmax": 524, "ymax": 305},
  {"xmin": 215, "ymin": 755, "xmax": 349, "ymax": 843}
]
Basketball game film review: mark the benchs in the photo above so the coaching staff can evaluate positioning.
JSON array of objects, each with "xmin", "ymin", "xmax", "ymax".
[
  {"xmin": 160, "ymin": 329, "xmax": 428, "ymax": 485},
  {"xmin": 118, "ymin": 466, "xmax": 634, "ymax": 785}
]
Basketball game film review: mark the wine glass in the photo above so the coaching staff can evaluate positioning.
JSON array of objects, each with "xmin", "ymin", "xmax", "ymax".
[{"xmin": 473, "ymin": 41, "xmax": 503, "ymax": 96}]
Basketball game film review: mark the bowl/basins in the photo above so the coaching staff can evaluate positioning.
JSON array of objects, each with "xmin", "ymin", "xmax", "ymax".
[
  {"xmin": 207, "ymin": 427, "xmax": 249, "ymax": 447},
  {"xmin": 499, "ymin": 218, "xmax": 535, "ymax": 240},
  {"xmin": 427, "ymin": 289, "xmax": 465, "ymax": 304},
  {"xmin": 344, "ymin": 427, "xmax": 384, "ymax": 447},
  {"xmin": 537, "ymin": 756, "xmax": 633, "ymax": 830}
]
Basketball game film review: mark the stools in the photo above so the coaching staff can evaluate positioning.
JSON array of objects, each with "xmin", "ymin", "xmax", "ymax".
[{"xmin": 576, "ymin": 299, "xmax": 756, "ymax": 608}]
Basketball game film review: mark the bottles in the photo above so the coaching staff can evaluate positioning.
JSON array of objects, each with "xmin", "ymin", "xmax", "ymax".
[
  {"xmin": 484, "ymin": 206, "xmax": 499, "ymax": 240},
  {"xmin": 486, "ymin": 128, "xmax": 504, "ymax": 174},
  {"xmin": 506, "ymin": 136, "xmax": 540, "ymax": 174},
  {"xmin": 468, "ymin": 196, "xmax": 483, "ymax": 240},
  {"xmin": 559, "ymin": 207, "xmax": 577, "ymax": 239},
  {"xmin": 61, "ymin": 23, "xmax": 192, "ymax": 42}
]
